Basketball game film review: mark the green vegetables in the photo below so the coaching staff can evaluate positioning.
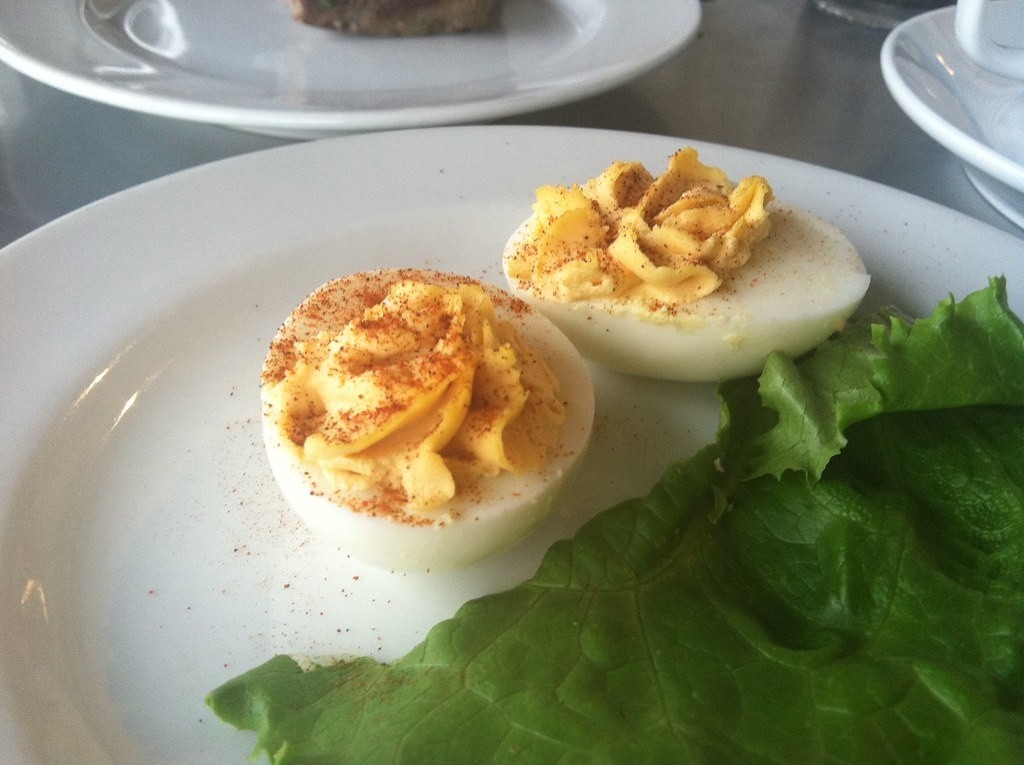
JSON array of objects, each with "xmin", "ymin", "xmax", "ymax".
[{"xmin": 203, "ymin": 279, "xmax": 1024, "ymax": 765}]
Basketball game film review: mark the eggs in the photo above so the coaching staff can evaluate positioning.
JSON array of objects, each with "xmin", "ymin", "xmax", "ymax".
[
  {"xmin": 259, "ymin": 267, "xmax": 592, "ymax": 569},
  {"xmin": 503, "ymin": 209, "xmax": 869, "ymax": 379}
]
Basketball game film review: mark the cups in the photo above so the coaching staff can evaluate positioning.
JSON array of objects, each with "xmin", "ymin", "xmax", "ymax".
[{"xmin": 955, "ymin": 0, "xmax": 1024, "ymax": 79}]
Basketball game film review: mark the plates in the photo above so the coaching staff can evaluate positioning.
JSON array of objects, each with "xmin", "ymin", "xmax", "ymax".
[
  {"xmin": 882, "ymin": 0, "xmax": 1024, "ymax": 231},
  {"xmin": 0, "ymin": 126, "xmax": 1024, "ymax": 765},
  {"xmin": 0, "ymin": 0, "xmax": 703, "ymax": 140}
]
